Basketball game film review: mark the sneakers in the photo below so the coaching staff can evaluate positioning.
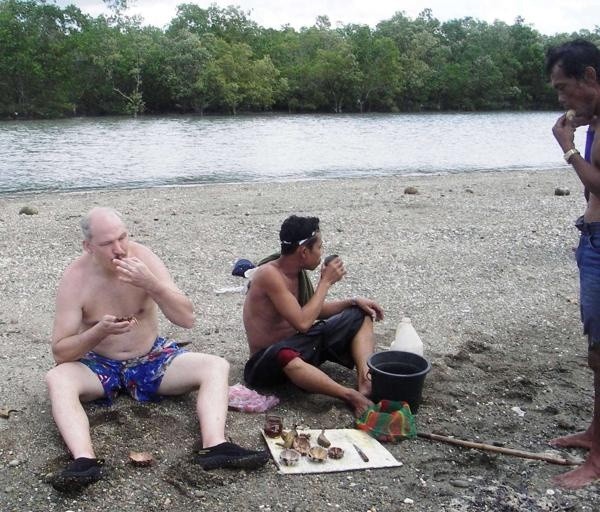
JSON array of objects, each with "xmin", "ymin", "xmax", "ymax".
[
  {"xmin": 195, "ymin": 441, "xmax": 270, "ymax": 471},
  {"xmin": 52, "ymin": 457, "xmax": 102, "ymax": 493}
]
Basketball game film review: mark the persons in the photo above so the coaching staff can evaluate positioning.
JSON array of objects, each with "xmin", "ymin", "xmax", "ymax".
[
  {"xmin": 43, "ymin": 204, "xmax": 269, "ymax": 494},
  {"xmin": 241, "ymin": 214, "xmax": 387, "ymax": 418},
  {"xmin": 545, "ymin": 40, "xmax": 600, "ymax": 492}
]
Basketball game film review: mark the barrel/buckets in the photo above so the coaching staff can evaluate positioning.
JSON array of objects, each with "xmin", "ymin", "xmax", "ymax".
[
  {"xmin": 388, "ymin": 317, "xmax": 424, "ymax": 359},
  {"xmin": 365, "ymin": 349, "xmax": 433, "ymax": 416}
]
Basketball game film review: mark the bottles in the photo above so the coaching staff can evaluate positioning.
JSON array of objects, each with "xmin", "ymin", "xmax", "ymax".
[{"xmin": 265, "ymin": 414, "xmax": 283, "ymax": 438}]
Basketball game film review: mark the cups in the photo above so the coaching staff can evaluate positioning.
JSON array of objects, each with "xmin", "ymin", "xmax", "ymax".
[{"xmin": 324, "ymin": 254, "xmax": 339, "ymax": 267}]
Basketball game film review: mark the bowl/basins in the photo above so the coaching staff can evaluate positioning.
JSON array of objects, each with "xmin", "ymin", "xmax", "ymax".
[
  {"xmin": 279, "ymin": 449, "xmax": 300, "ymax": 467},
  {"xmin": 128, "ymin": 450, "xmax": 154, "ymax": 466},
  {"xmin": 307, "ymin": 445, "xmax": 327, "ymax": 464}
]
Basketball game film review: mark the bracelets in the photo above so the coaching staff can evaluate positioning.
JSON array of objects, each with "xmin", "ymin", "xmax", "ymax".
[{"xmin": 562, "ymin": 146, "xmax": 581, "ymax": 166}]
